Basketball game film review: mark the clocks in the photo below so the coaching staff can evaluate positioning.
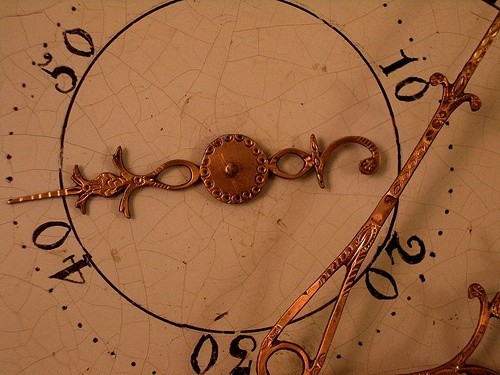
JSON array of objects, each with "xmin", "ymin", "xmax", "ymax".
[{"xmin": 0, "ymin": 0, "xmax": 499, "ymax": 373}]
